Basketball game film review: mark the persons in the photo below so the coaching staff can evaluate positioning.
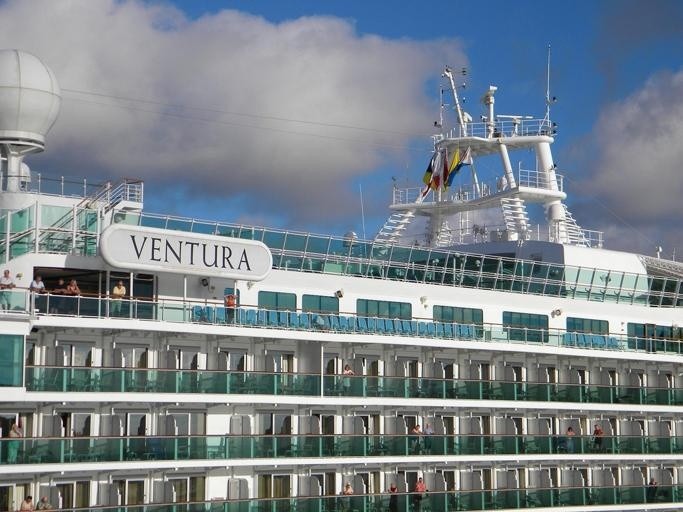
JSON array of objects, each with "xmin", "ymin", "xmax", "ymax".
[
  {"xmin": 29, "ymin": 275, "xmax": 45, "ymax": 311},
  {"xmin": 565, "ymin": 426, "xmax": 576, "ymax": 453},
  {"xmin": 343, "ymin": 481, "xmax": 353, "ymax": 512},
  {"xmin": 592, "ymin": 424, "xmax": 603, "ymax": 453},
  {"xmin": 20, "ymin": 496, "xmax": 51, "ymax": 511},
  {"xmin": 411, "ymin": 425, "xmax": 422, "ymax": 453},
  {"xmin": 110, "ymin": 280, "xmax": 126, "ymax": 316},
  {"xmin": 414, "ymin": 477, "xmax": 425, "ymax": 511},
  {"xmin": 424, "ymin": 424, "xmax": 434, "ymax": 455},
  {"xmin": 648, "ymin": 478, "xmax": 658, "ymax": 502},
  {"xmin": 0, "ymin": 269, "xmax": 17, "ymax": 310},
  {"xmin": 340, "ymin": 364, "xmax": 353, "ymax": 395},
  {"xmin": 51, "ymin": 278, "xmax": 81, "ymax": 314},
  {"xmin": 7, "ymin": 424, "xmax": 21, "ymax": 464},
  {"xmin": 387, "ymin": 483, "xmax": 398, "ymax": 511},
  {"xmin": 222, "ymin": 293, "xmax": 238, "ymax": 324}
]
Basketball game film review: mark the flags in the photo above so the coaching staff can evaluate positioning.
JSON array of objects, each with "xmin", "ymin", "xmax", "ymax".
[{"xmin": 423, "ymin": 145, "xmax": 472, "ymax": 193}]
